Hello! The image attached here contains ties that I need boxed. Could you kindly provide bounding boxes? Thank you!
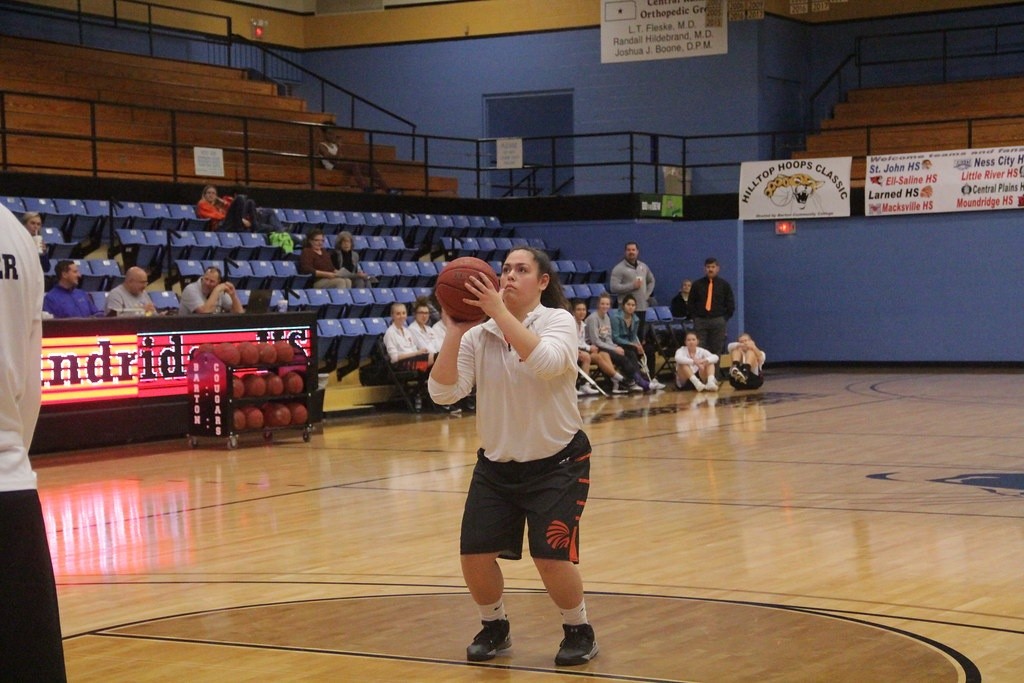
[706,278,713,311]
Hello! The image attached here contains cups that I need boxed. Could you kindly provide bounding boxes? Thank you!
[278,300,288,313]
[33,235,43,255]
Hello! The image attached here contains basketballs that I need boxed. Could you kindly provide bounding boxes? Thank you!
[435,256,499,323]
[199,341,294,366]
[233,400,308,430]
[232,372,304,397]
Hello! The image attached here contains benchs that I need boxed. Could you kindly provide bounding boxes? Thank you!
[790,74,1024,189]
[0,36,461,196]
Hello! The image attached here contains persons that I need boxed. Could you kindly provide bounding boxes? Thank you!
[196,184,284,233]
[318,121,404,195]
[727,333,766,390]
[675,331,719,392]
[20,211,51,273]
[104,267,158,317]
[298,229,370,289]
[178,267,244,315]
[383,299,475,414]
[428,244,600,666]
[610,243,655,345]
[42,260,104,318]
[572,292,666,396]
[670,258,735,387]
[0,201,69,683]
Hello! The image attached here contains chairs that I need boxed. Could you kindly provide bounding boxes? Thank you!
[0,195,694,413]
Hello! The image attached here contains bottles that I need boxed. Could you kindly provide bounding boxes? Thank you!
[415,394,422,413]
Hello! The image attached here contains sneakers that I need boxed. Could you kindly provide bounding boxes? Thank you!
[467,614,511,662]
[554,623,599,666]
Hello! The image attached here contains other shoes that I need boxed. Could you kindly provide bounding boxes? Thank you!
[735,371,746,386]
[612,385,628,393]
[464,399,475,409]
[629,383,643,391]
[620,377,635,387]
[705,381,718,392]
[651,378,665,389]
[579,382,599,395]
[696,381,705,392]
[648,381,658,390]
[444,405,462,413]
[575,391,585,396]
[730,366,739,378]
